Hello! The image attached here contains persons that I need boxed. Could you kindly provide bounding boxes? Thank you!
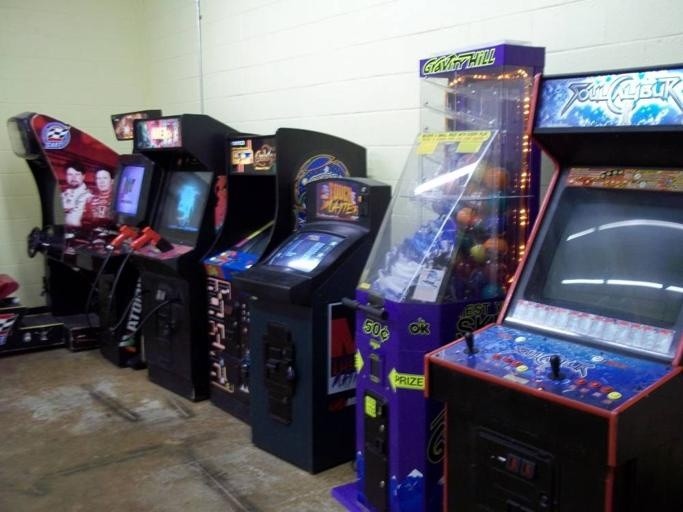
[61,159,93,227]
[81,167,114,226]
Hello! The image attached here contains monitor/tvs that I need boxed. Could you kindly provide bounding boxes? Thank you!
[260,224,364,281]
[51,179,65,225]
[109,163,149,228]
[519,183,683,331]
[152,168,214,246]
